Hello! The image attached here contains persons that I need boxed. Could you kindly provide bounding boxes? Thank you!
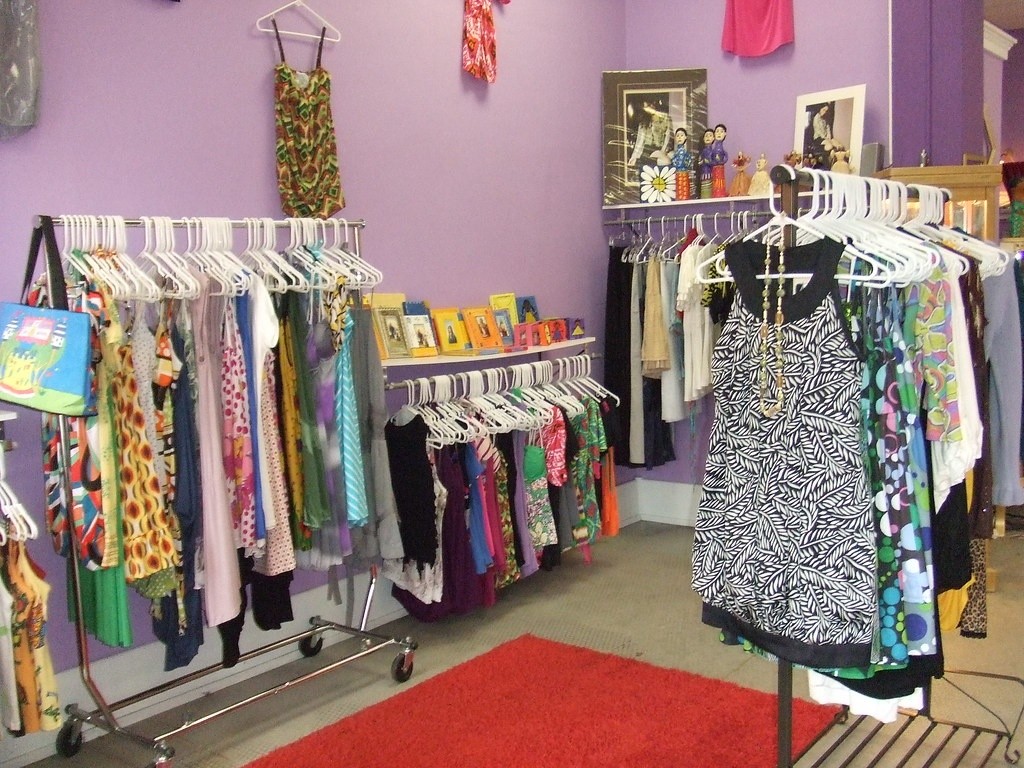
[748,152,774,194]
[627,93,674,168]
[448,325,457,343]
[730,150,753,196]
[674,128,691,201]
[811,102,844,170]
[697,124,728,199]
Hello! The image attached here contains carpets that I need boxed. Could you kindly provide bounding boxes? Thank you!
[243,629,853,768]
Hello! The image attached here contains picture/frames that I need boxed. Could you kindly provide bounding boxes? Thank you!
[599,67,710,201]
[792,85,868,186]
[365,291,586,359]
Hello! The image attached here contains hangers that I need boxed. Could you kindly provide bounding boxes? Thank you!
[46,215,383,304]
[0,443,40,548]
[257,2,343,43]
[607,156,1010,288]
[386,346,624,448]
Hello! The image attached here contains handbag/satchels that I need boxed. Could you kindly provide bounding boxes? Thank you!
[0,216,98,417]
